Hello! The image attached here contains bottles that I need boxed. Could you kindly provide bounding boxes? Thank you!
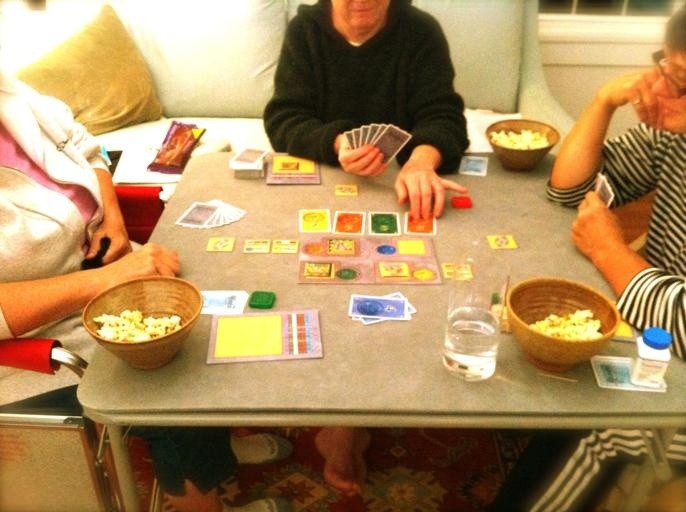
[629,328,672,387]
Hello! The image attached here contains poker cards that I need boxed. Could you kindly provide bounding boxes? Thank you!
[230,146,269,170]
[300,209,437,237]
[199,291,250,315]
[346,122,414,165]
[590,355,668,395]
[460,156,489,176]
[596,172,615,208]
[348,293,417,326]
[175,199,247,229]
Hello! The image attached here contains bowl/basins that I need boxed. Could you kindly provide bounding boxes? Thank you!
[486,118,560,172]
[81,275,204,369]
[505,275,620,373]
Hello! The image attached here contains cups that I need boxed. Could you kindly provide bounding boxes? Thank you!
[443,289,499,381]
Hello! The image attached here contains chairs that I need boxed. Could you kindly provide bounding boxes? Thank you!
[594,459,686,512]
[0,188,166,512]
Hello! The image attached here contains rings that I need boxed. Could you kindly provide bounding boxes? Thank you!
[633,99,644,106]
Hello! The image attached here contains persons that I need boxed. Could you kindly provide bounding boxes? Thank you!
[0,66,297,511]
[262,1,472,495]
[478,3,686,510]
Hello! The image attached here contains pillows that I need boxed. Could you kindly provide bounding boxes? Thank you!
[112,0,288,117]
[289,0,527,112]
[14,0,163,137]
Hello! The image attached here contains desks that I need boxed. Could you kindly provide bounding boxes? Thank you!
[74,151,686,512]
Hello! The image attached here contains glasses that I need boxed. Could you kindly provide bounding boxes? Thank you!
[652,48,685,100]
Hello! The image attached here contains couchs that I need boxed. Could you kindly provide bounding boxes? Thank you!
[0,0,578,203]
